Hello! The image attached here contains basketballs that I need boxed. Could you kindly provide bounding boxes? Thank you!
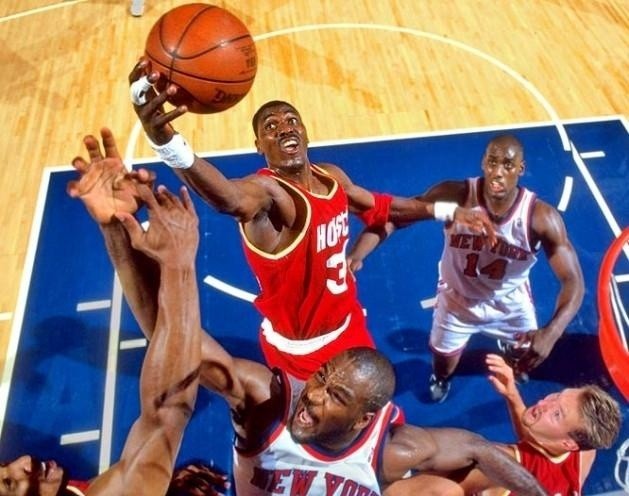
[145,4,257,114]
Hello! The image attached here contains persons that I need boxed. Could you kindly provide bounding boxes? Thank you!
[126,55,500,387]
[68,129,550,496]
[457,352,623,496]
[76,179,206,496]
[1,455,232,496]
[346,133,589,405]
[129,0,146,16]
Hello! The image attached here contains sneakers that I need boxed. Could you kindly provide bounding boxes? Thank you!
[496,339,529,384]
[428,371,451,403]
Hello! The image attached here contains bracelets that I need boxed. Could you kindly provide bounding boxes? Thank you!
[145,129,194,170]
[432,200,459,224]
